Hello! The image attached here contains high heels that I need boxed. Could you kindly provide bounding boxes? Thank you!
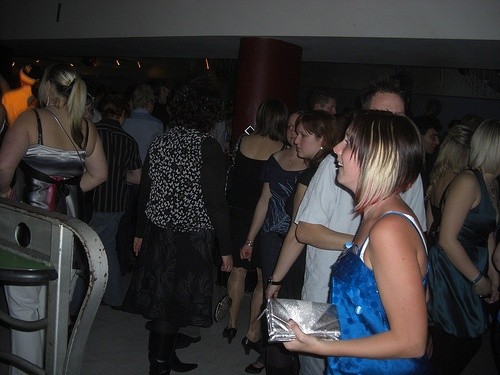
[241,337,262,355]
[223,326,237,344]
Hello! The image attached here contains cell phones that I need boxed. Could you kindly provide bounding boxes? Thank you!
[245,126,255,135]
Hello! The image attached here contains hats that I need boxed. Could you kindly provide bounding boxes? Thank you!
[20,64,42,85]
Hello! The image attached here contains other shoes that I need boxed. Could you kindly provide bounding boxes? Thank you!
[175,333,202,349]
[170,353,198,372]
[245,362,264,373]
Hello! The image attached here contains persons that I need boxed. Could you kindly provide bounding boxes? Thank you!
[294,78,427,374]
[239,107,310,375]
[305,87,338,115]
[284,110,436,374]
[132,75,233,374]
[425,116,500,375]
[0,46,233,284]
[0,60,108,375]
[411,114,440,154]
[414,99,444,124]
[420,116,485,240]
[67,89,140,348]
[224,95,293,355]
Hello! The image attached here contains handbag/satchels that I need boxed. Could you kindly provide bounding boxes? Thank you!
[215,270,232,322]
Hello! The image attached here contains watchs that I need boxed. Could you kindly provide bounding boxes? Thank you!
[267,276,282,285]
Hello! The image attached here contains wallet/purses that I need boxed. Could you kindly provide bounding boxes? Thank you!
[257,299,341,342]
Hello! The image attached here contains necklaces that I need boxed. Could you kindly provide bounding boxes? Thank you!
[489,190,497,198]
[344,193,398,249]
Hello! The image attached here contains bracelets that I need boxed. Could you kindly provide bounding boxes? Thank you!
[470,272,483,285]
